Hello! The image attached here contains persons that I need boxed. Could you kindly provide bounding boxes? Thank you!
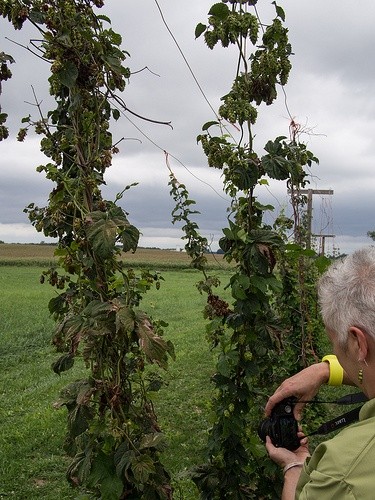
[263,243,375,500]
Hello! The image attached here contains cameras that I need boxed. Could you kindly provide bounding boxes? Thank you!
[257,395,301,452]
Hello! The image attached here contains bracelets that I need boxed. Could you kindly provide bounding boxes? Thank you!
[282,461,305,476]
[320,354,344,386]
